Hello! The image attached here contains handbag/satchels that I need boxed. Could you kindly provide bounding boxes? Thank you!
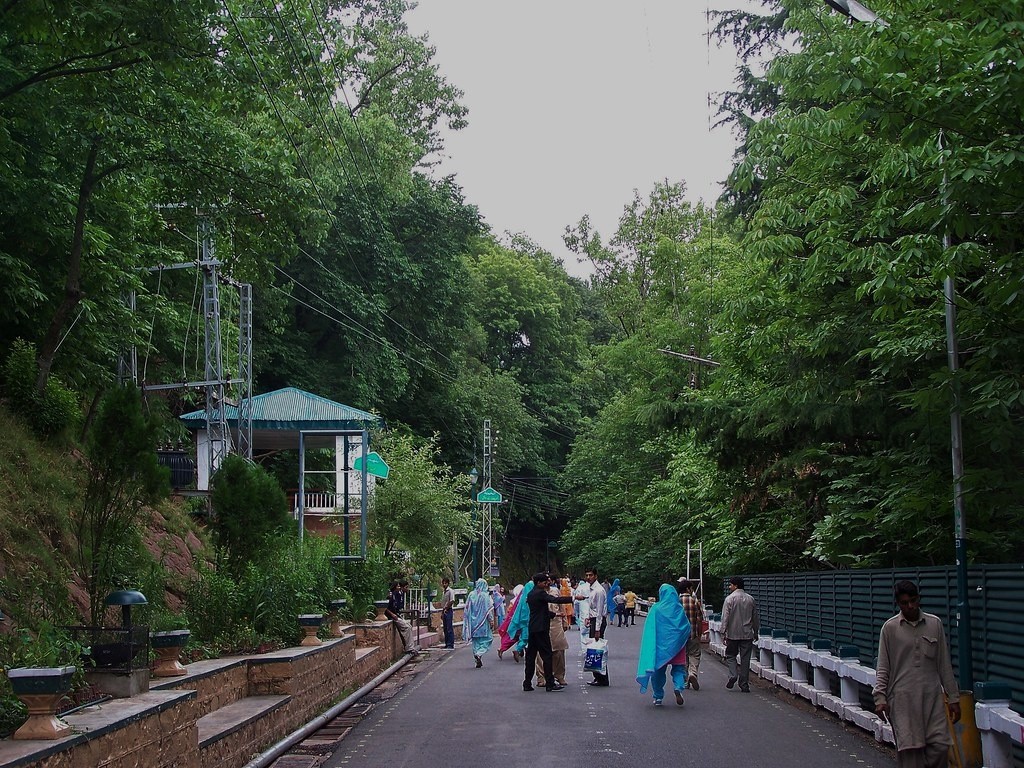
[582,637,608,675]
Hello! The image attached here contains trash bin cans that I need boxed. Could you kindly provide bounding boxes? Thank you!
[700,621,709,644]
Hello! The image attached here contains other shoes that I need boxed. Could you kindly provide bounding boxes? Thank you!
[523,687,534,691]
[689,674,699,691]
[610,621,636,627]
[653,699,662,705]
[440,647,454,650]
[552,684,565,691]
[726,679,735,689]
[537,681,546,687]
[512,650,519,663]
[520,649,524,657]
[554,673,567,685]
[742,687,750,693]
[685,683,690,689]
[474,654,483,669]
[587,681,599,686]
[674,689,684,705]
[497,650,503,660]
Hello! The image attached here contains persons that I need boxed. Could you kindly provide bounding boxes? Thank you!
[674,580,704,692]
[873,582,962,768]
[440,578,456,650]
[721,577,759,692]
[465,567,642,693]
[385,581,420,655]
[643,583,690,707]
